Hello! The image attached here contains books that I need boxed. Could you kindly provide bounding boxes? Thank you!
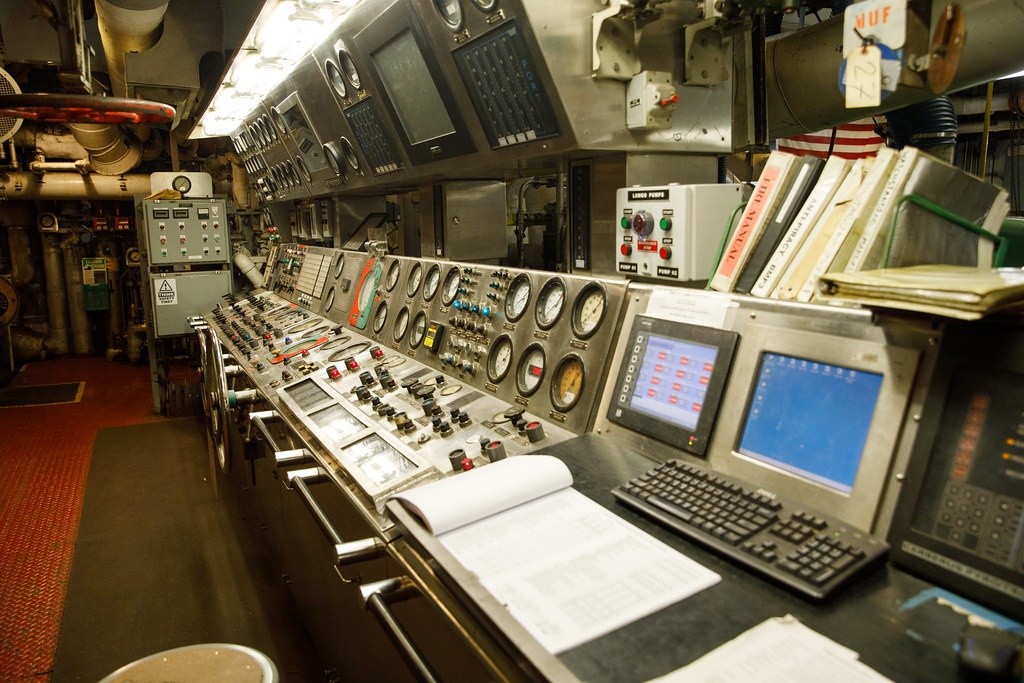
[711,149,1024,321]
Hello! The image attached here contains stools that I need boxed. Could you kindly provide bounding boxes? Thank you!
[97,643,279,683]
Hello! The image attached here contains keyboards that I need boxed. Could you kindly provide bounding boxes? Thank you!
[610,458,891,600]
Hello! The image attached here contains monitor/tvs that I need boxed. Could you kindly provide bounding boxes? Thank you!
[606,314,739,455]
[708,323,921,534]
